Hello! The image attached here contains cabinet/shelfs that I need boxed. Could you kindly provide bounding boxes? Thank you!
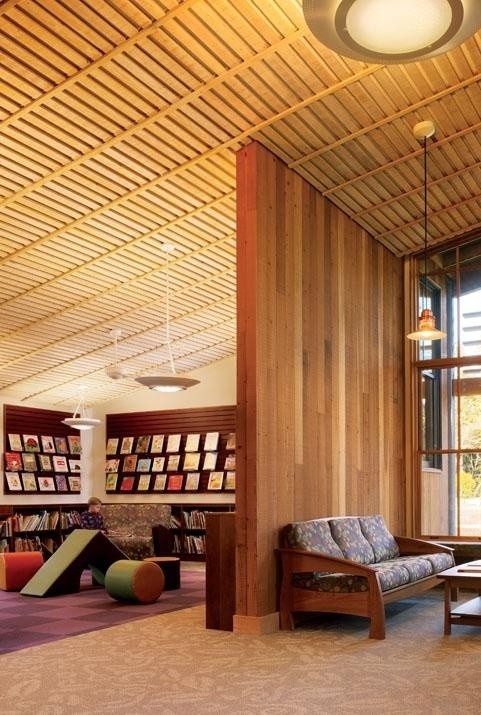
[170,503,236,561]
[0,503,87,565]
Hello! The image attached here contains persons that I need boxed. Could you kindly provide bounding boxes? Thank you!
[81,496,109,534]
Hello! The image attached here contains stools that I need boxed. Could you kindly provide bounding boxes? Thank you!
[144,557,182,589]
[105,559,166,606]
[1,551,45,591]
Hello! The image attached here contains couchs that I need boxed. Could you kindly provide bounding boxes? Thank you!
[97,504,171,560]
[273,517,459,641]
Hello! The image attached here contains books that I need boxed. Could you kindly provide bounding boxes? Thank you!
[6,432,236,492]
[169,504,211,555]
[0,505,80,552]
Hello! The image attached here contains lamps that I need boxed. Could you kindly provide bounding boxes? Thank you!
[405,121,449,340]
[302,1,480,67]
[134,243,201,394]
[106,329,128,381]
[61,383,100,430]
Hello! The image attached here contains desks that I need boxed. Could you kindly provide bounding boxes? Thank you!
[436,557,481,639]
[206,510,237,632]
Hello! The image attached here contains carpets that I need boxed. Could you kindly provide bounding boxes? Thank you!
[0,566,206,654]
